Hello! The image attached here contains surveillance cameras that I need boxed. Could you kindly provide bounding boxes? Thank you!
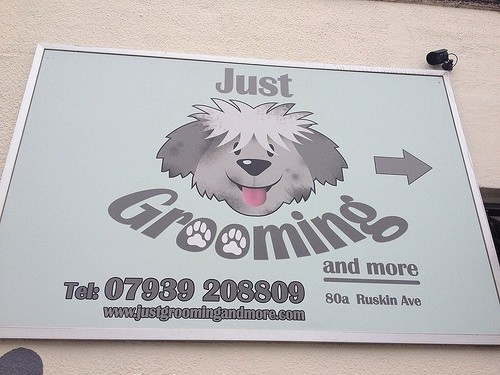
[426,48,454,71]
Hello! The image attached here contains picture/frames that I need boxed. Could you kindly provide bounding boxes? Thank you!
[0,43,500,347]
